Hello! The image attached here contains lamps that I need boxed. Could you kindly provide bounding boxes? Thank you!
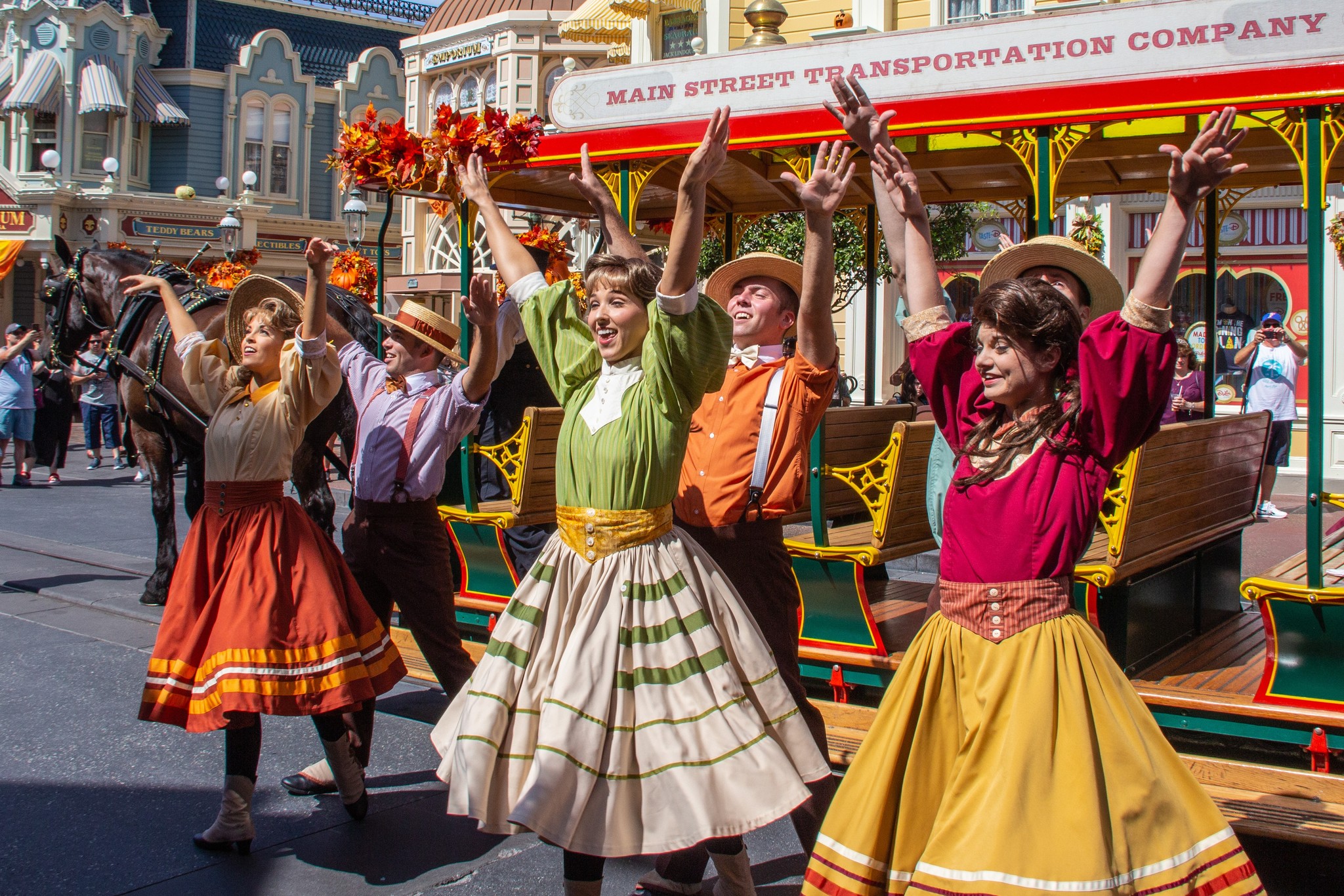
[41,149,61,174]
[102,157,119,178]
[215,176,230,196]
[242,170,257,190]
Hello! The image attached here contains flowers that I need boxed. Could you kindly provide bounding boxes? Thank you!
[328,247,377,304]
[321,99,587,315]
[106,240,263,287]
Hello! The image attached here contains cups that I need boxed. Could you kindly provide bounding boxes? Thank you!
[1170,393,1182,412]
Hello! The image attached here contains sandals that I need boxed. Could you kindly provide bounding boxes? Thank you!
[20,471,32,481]
[49,472,62,484]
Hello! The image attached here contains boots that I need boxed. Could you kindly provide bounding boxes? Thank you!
[193,773,259,855]
[704,833,756,896]
[318,726,371,822]
[563,876,604,896]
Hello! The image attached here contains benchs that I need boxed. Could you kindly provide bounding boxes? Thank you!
[436,407,565,603]
[1069,409,1273,677]
[1240,490,1344,712]
[782,401,917,525]
[783,421,936,656]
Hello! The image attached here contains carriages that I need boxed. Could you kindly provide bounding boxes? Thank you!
[37,0,1344,850]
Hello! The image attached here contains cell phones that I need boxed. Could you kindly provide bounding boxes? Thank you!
[32,323,40,333]
[1261,331,1278,339]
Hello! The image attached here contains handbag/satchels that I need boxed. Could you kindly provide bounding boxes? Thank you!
[33,386,44,409]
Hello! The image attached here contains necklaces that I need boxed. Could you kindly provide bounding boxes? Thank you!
[1174,379,1181,386]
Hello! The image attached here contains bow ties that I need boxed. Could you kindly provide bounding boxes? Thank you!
[728,345,761,370]
[386,374,408,394]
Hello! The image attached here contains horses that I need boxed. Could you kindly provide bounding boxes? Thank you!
[39,233,390,604]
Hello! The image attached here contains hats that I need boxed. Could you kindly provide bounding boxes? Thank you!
[6,323,27,334]
[976,233,1126,336]
[1262,312,1282,323]
[224,273,305,367]
[371,299,469,366]
[489,243,550,278]
[704,250,807,339]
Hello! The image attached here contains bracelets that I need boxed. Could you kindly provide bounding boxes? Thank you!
[1189,402,1194,416]
[1285,339,1292,343]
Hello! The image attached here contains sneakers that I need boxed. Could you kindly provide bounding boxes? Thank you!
[12,475,32,488]
[1256,501,1288,519]
[87,458,102,470]
[133,469,149,483]
[112,458,124,469]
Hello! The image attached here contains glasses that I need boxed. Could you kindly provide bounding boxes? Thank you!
[10,330,25,336]
[89,339,102,344]
[1263,322,1279,329]
[782,352,795,358]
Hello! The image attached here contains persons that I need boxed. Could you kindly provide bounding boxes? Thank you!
[122,238,367,846]
[431,105,830,896]
[569,139,855,896]
[21,327,74,484]
[281,275,501,797]
[1234,312,1307,519]
[71,330,150,483]
[0,323,44,487]
[794,127,1274,896]
[821,73,1249,628]
[1160,335,1218,425]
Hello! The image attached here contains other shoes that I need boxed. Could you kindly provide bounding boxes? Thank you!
[119,448,127,456]
[281,772,368,795]
[87,451,102,460]
[633,882,703,896]
